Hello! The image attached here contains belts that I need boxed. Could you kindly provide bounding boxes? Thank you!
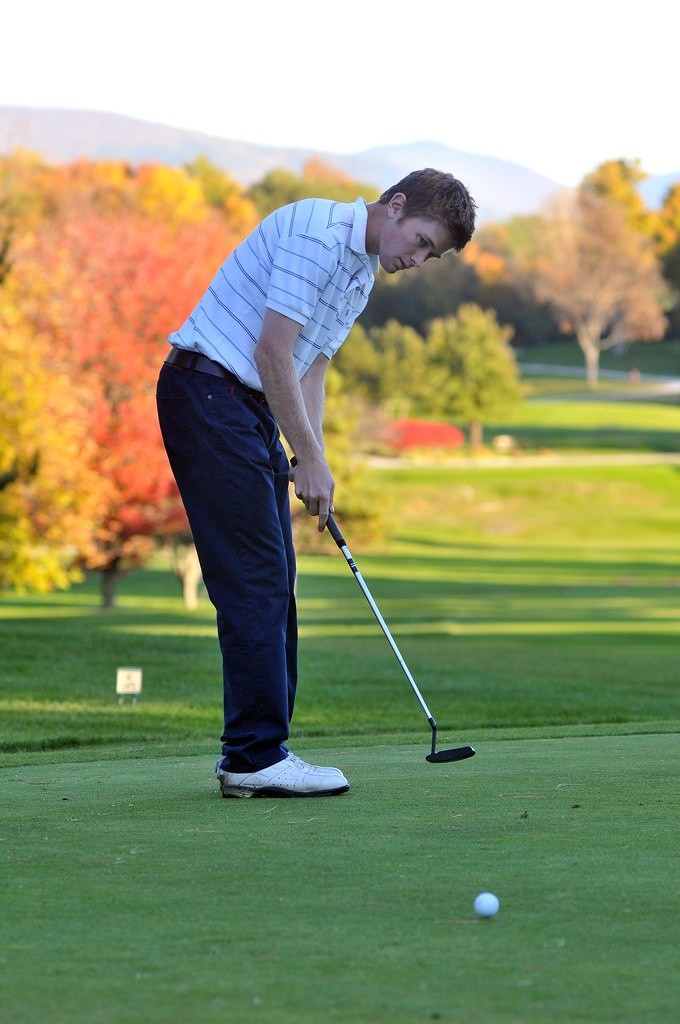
[163,348,235,380]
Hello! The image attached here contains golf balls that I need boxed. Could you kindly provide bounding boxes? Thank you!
[474,893,499,919]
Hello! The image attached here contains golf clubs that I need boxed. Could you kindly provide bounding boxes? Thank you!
[286,450,479,763]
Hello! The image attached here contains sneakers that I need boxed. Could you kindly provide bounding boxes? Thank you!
[215,750,349,798]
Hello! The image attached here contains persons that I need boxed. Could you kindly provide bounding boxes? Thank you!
[153,167,480,797]
